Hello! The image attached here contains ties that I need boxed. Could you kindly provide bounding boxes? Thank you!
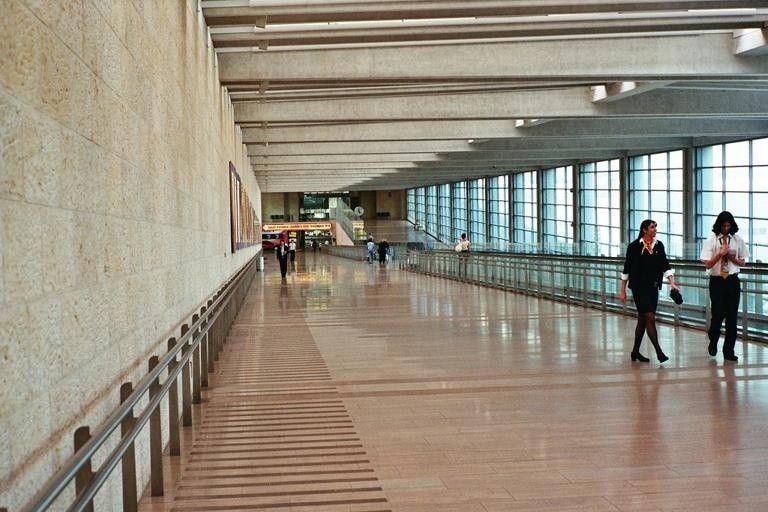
[719,236,730,279]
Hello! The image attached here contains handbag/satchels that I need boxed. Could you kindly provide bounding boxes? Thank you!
[455,241,463,253]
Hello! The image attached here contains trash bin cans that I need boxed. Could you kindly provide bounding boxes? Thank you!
[256,257,264,271]
[407,242,425,268]
[413,224,420,231]
[387,247,395,260]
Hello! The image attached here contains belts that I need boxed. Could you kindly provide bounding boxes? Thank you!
[709,272,739,279]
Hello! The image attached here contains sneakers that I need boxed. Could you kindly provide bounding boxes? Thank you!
[631,352,651,362]
[723,350,739,361]
[708,340,718,356]
[657,354,670,363]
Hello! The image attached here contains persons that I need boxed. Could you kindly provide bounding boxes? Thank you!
[619,219,680,363]
[277,240,290,278]
[407,246,420,270]
[700,211,751,361]
[310,239,317,253]
[458,233,471,277]
[324,239,330,246]
[367,238,376,264]
[378,239,387,265]
[288,240,297,261]
